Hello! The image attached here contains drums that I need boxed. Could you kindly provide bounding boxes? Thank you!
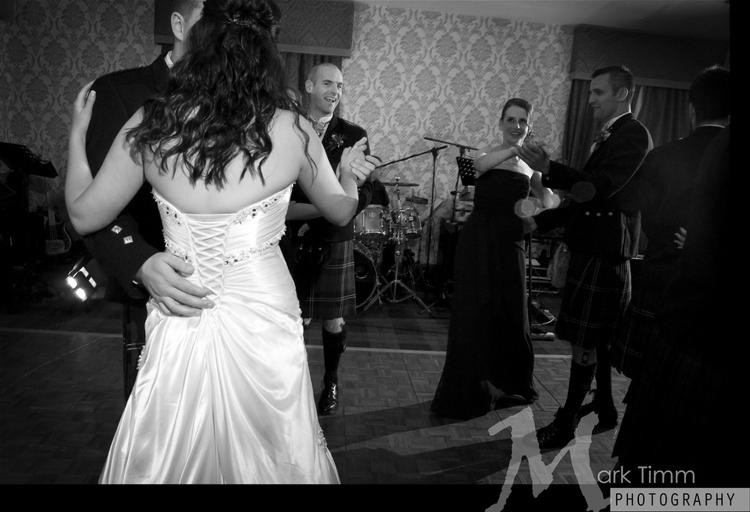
[353,238,377,308]
[352,204,390,240]
[391,207,422,240]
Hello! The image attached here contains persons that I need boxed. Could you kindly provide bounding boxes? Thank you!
[599,67,731,496]
[355,156,390,206]
[78,0,207,400]
[430,98,556,421]
[65,0,366,486]
[513,63,654,450]
[671,125,731,300]
[279,62,369,416]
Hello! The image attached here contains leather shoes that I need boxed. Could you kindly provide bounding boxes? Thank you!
[522,406,577,454]
[575,398,618,427]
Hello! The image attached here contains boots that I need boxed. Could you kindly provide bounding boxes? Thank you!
[318,327,347,415]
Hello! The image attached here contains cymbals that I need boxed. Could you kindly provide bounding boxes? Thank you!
[382,183,420,186]
